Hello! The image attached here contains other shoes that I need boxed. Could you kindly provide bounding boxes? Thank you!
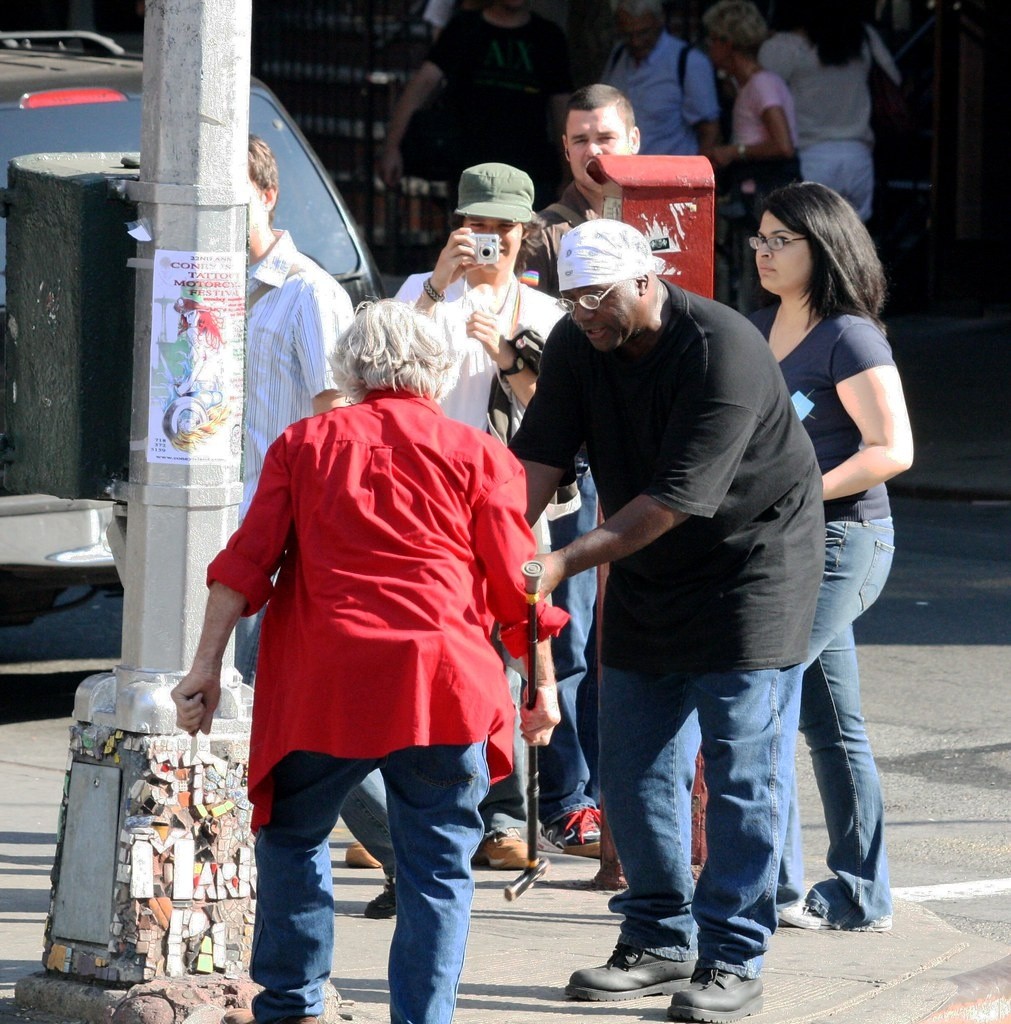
[778,903,893,933]
[220,1009,320,1024]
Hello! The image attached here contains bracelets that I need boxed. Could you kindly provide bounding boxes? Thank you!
[423,277,445,302]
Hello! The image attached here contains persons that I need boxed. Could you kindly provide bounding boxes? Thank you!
[171,300,568,1024]
[508,217,825,1024]
[736,182,914,935]
[595,0,899,315]
[342,163,582,870]
[235,133,404,920]
[511,78,644,858]
[375,0,582,212]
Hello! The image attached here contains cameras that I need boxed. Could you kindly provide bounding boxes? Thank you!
[461,234,500,264]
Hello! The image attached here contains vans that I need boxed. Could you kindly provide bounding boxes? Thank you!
[0,29,391,687]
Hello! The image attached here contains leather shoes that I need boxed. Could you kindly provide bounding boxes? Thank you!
[666,968,763,1023]
[564,945,697,1001]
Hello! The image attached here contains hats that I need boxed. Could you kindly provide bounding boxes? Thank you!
[455,163,534,223]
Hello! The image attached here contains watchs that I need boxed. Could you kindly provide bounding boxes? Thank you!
[737,144,745,160]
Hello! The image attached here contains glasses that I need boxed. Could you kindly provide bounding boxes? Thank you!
[749,236,809,250]
[556,282,618,314]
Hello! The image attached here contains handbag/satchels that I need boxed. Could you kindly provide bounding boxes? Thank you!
[867,39,921,152]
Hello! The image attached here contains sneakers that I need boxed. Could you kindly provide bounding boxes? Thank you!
[537,806,601,858]
[345,841,382,868]
[473,828,528,870]
[364,878,396,918]
